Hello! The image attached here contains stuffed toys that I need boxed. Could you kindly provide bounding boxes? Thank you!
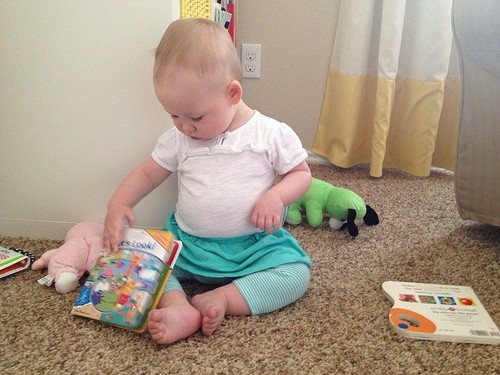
[31,220,105,293]
[285,177,380,238]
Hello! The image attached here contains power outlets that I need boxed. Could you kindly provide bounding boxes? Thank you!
[241,43,262,80]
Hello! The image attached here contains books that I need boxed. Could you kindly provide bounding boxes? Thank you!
[0,246,28,279]
[382,281,500,345]
[70,228,182,333]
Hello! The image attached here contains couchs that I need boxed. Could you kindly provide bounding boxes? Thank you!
[453,23,500,228]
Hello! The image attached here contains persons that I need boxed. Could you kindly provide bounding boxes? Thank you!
[103,18,312,344]
